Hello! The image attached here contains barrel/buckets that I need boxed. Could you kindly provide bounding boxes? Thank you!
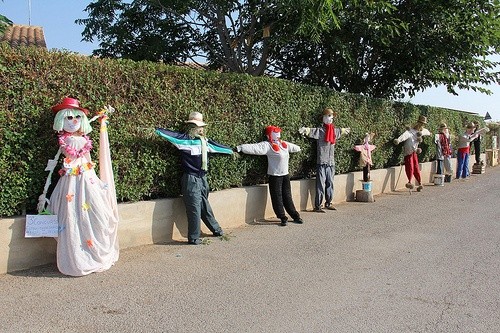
[362,182,372,191]
[433,174,445,187]
